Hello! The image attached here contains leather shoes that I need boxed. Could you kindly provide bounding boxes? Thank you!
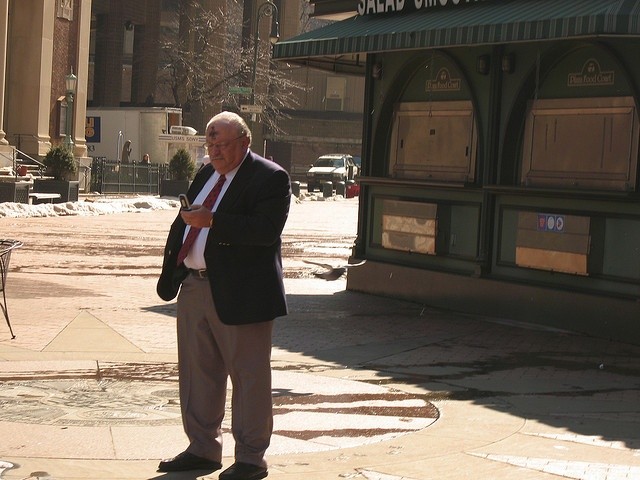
[219,462,268,480]
[158,449,222,471]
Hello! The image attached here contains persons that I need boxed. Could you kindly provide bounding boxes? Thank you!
[122,140,132,163]
[156,111,291,480]
[141,153,150,164]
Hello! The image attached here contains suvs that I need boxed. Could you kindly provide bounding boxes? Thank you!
[307,153,360,192]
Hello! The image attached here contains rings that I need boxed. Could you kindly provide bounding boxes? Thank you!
[186,219,193,226]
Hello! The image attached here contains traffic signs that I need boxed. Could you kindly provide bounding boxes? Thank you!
[228,86,252,94]
[240,105,262,114]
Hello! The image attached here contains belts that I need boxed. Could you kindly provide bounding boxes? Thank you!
[182,266,208,279]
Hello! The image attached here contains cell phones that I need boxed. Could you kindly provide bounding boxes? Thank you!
[179,194,192,210]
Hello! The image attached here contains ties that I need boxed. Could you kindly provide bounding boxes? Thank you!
[176,174,226,267]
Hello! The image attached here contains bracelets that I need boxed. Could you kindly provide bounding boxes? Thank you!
[209,216,214,230]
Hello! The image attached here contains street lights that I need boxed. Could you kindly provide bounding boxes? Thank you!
[249,2,278,132]
[65,65,77,154]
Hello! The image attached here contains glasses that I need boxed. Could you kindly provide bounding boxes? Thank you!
[200,133,246,151]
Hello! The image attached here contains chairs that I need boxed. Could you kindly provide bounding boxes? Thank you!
[1,239,24,339]
